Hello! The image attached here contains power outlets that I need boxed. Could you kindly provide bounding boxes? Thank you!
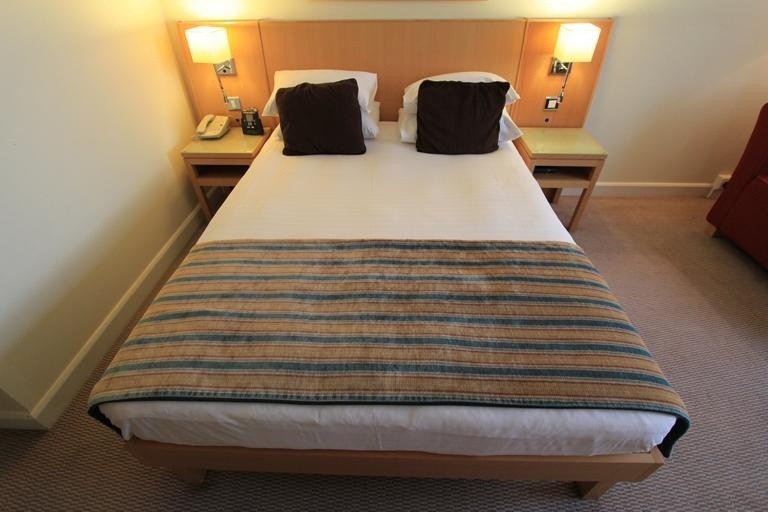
[714,173,735,192]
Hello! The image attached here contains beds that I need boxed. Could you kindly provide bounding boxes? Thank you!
[85,120,691,500]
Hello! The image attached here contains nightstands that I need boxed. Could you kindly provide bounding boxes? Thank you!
[515,127,608,232]
[179,126,271,222]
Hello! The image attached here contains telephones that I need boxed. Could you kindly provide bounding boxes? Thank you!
[191,114,229,141]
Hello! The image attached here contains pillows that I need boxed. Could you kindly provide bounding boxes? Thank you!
[275,78,366,155]
[403,71,520,113]
[398,108,524,143]
[415,79,510,154]
[261,69,378,116]
[274,101,380,139]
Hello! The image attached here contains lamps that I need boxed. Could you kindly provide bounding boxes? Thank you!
[185,25,236,104]
[547,22,601,102]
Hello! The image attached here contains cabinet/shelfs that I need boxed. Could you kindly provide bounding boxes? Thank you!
[706,105,768,272]
